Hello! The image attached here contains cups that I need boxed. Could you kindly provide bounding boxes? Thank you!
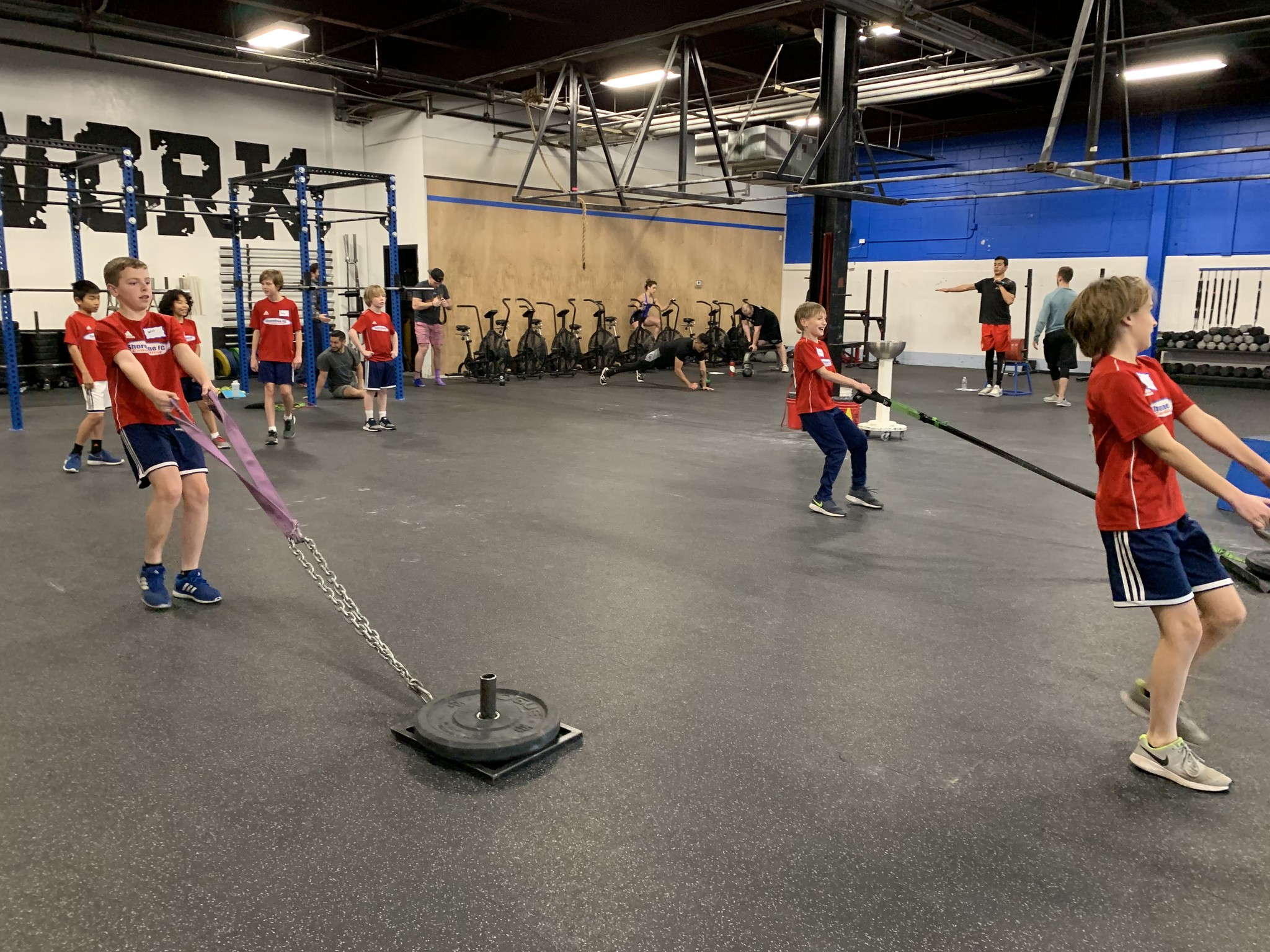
[231,380,240,400]
[499,368,505,386]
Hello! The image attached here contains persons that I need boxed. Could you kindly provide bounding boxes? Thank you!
[94,256,224,609]
[302,330,379,398]
[294,262,331,388]
[792,301,884,518]
[348,284,399,432]
[629,279,675,344]
[740,302,789,373]
[600,333,715,390]
[249,270,302,446]
[1032,267,1079,407]
[158,289,232,450]
[413,267,452,387]
[61,280,125,473]
[1065,274,1270,793]
[935,255,1016,397]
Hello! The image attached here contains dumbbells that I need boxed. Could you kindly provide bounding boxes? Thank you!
[741,361,754,377]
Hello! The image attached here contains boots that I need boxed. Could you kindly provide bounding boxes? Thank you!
[435,369,446,386]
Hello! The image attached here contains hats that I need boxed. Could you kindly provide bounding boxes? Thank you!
[426,268,444,283]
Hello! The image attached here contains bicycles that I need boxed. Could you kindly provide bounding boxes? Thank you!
[450,298,797,382]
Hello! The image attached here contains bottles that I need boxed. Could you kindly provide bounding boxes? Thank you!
[962,377,967,391]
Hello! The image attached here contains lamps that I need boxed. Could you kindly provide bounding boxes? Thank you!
[600,64,682,88]
[245,21,311,49]
[814,28,823,44]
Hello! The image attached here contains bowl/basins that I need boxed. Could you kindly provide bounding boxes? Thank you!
[866,340,906,359]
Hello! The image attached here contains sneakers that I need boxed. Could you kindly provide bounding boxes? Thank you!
[86,449,125,465]
[172,568,220,603]
[781,365,789,373]
[846,485,882,510]
[62,452,81,472]
[377,416,396,430]
[1043,394,1060,403]
[808,493,848,518]
[635,370,645,382]
[1128,730,1234,794]
[600,367,611,385]
[134,566,171,609]
[988,384,1003,397]
[978,384,991,396]
[414,378,426,387]
[283,414,296,439]
[265,430,278,444]
[363,417,382,431]
[1056,398,1072,407]
[210,436,232,449]
[1118,677,1211,746]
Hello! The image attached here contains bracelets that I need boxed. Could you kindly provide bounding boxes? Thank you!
[431,302,434,306]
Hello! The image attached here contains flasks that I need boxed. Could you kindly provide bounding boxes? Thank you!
[729,358,735,377]
